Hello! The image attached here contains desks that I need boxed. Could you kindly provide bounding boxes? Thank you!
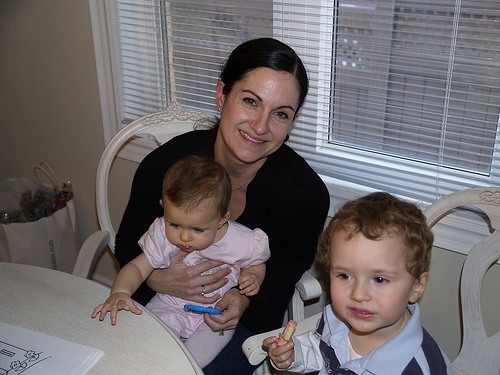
[0,262,205,374]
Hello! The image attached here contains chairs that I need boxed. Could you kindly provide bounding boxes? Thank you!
[240,187,500,374]
[71,106,219,278]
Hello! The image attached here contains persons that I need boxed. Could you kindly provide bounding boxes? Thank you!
[114,38,331,375]
[91,157,270,370]
[263,192,452,375]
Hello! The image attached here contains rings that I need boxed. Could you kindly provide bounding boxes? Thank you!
[202,285,206,296]
[219,329,224,336]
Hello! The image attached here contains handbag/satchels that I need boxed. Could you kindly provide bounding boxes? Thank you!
[3,161,81,274]
